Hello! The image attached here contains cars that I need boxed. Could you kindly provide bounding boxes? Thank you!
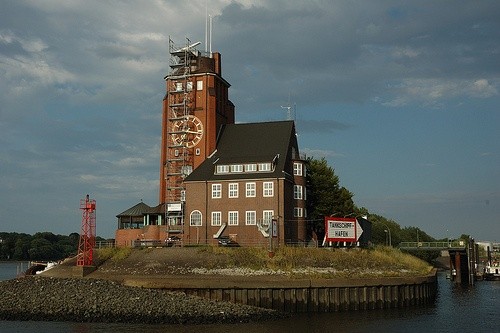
[165,236,182,243]
[218,235,233,247]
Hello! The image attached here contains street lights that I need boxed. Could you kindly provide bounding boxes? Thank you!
[416,226,419,247]
[383,230,389,245]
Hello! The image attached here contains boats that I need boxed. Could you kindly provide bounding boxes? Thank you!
[484,262,500,281]
[446,274,451,279]
